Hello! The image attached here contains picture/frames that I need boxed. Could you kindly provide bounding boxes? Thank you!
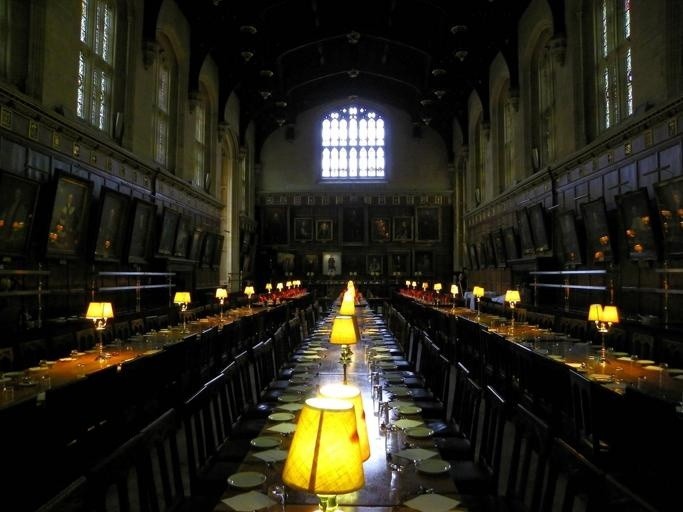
[0,167,215,269]
[468,175,682,270]
[259,203,443,280]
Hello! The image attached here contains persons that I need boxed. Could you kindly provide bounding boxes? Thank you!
[0,188,117,267]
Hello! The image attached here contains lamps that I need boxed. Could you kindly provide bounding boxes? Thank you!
[215,288,228,319]
[172,291,193,335]
[240,24,467,125]
[277,282,283,294]
[504,290,521,325]
[587,303,619,351]
[244,286,255,309]
[84,301,114,359]
[473,286,485,312]
[285,280,301,291]
[434,283,442,294]
[422,282,428,291]
[405,281,411,288]
[328,281,360,380]
[412,282,417,290]
[265,283,273,295]
[450,285,458,304]
[281,382,373,511]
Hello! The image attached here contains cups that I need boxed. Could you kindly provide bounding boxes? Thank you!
[3,385,14,400]
[38,375,49,389]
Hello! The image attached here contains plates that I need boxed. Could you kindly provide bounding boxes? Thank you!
[249,436,282,448]
[268,412,295,421]
[227,471,266,490]
[278,394,302,402]
[415,290,670,402]
[399,405,422,415]
[0,303,251,386]
[415,458,451,476]
[406,427,434,438]
[282,290,413,395]
